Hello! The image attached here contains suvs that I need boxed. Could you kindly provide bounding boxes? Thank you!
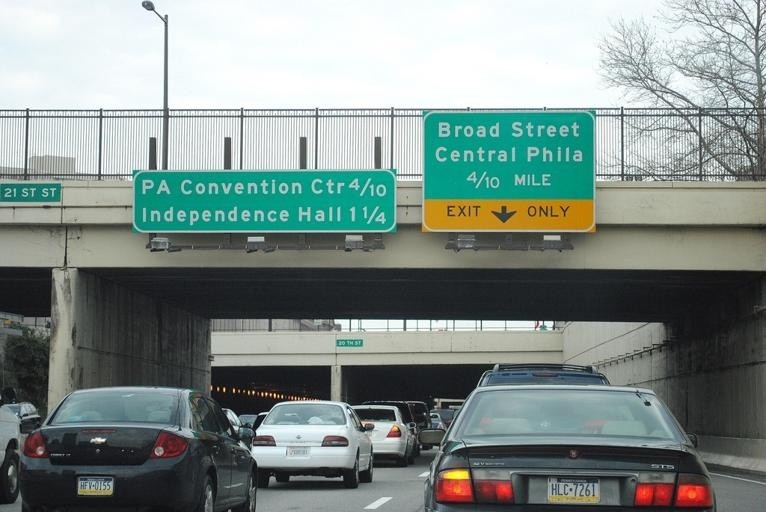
[4,401,41,433]
[0,395,20,503]
[475,363,612,386]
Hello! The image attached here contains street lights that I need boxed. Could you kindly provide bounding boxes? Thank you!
[141,0,169,170]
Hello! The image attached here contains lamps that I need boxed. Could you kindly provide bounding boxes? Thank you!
[150,237,173,252]
[456,233,476,250]
[246,236,266,253]
[345,234,364,252]
[543,234,564,249]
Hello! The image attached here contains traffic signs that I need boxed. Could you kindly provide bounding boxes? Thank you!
[424,110,597,234]
[0,182,61,203]
[131,166,400,237]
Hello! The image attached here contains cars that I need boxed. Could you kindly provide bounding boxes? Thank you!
[416,385,717,511]
[219,408,241,439]
[405,400,434,450]
[433,408,455,430]
[423,412,447,441]
[250,400,374,489]
[350,405,416,467]
[19,388,258,510]
[361,400,420,456]
[254,410,268,434]
[237,414,255,428]
